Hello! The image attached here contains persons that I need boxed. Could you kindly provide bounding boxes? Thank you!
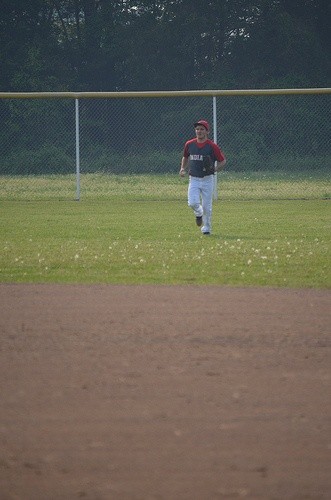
[179,119,226,233]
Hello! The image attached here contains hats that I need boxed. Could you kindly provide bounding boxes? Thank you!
[194,120,210,130]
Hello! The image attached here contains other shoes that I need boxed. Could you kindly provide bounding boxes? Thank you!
[201,224,210,233]
[196,216,203,226]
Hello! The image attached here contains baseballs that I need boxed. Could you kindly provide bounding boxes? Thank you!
[182,171,186,175]
